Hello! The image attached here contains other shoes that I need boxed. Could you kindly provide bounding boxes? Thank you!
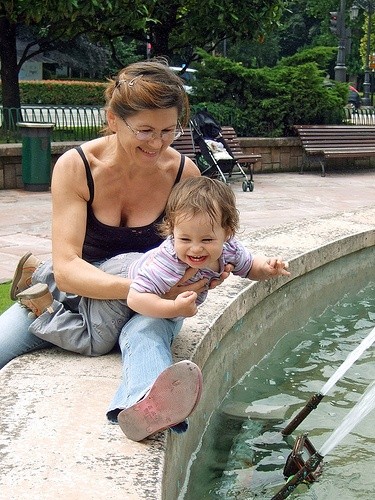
[117,360,203,442]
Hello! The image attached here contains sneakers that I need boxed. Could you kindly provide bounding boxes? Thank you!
[10,250,42,301]
[16,283,54,316]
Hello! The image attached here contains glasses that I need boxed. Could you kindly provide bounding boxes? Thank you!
[121,116,184,142]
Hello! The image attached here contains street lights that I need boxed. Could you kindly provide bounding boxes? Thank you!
[350,0,375,106]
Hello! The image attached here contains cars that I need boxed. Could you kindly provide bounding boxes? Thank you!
[349,86,360,115]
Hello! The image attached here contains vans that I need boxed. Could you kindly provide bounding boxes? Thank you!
[170,67,197,95]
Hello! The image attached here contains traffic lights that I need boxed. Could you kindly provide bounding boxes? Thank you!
[328,7,340,39]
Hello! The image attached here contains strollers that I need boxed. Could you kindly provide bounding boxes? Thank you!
[189,111,254,192]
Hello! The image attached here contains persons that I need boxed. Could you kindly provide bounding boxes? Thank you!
[10,176,292,356]
[1,59,233,442]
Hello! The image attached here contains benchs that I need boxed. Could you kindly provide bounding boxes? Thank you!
[171,126,263,182]
[289,125,375,177]
